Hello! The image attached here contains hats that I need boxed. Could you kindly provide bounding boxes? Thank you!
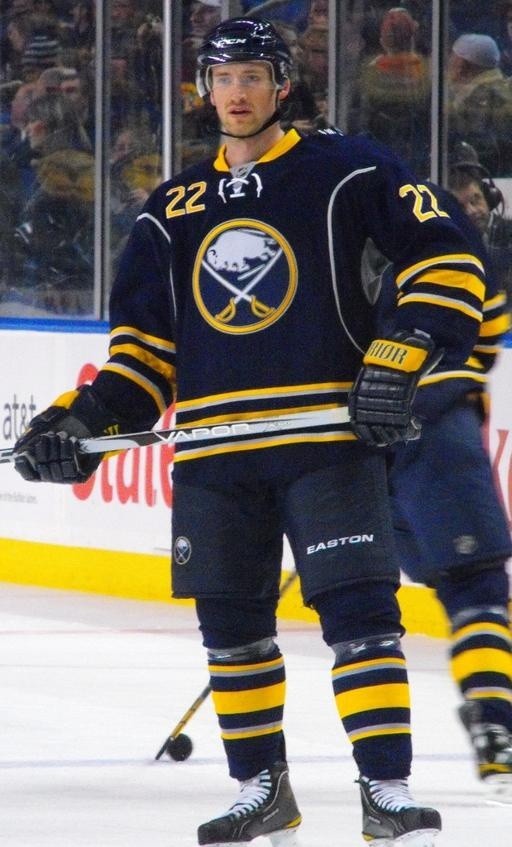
[454,32,501,69]
[381,8,419,44]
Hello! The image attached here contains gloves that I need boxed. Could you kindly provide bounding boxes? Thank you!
[347,328,446,448]
[12,383,129,483]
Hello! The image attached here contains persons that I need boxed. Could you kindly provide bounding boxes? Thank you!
[311,217,511,788]
[2,1,512,335]
[3,15,494,847]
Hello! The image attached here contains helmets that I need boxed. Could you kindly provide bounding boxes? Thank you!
[196,15,294,97]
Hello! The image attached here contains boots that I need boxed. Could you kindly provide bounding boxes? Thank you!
[459,699,510,778]
[358,771,442,840]
[198,759,301,844]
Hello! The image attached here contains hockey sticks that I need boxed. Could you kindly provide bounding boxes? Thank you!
[153,567,299,765]
